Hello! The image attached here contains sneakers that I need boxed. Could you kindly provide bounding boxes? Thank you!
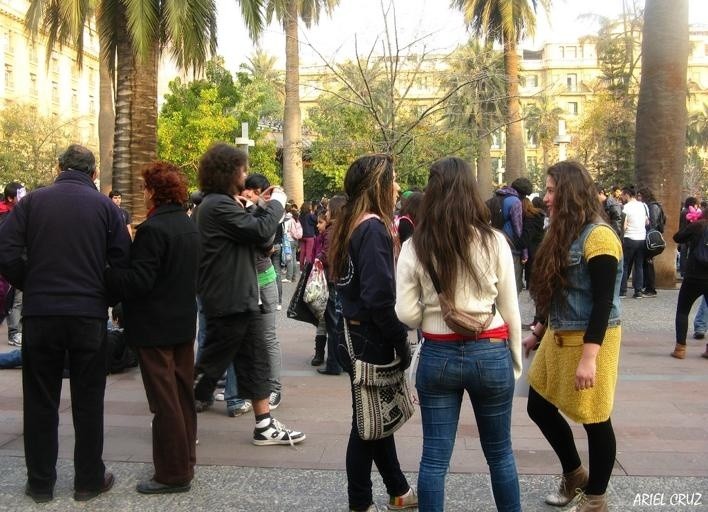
[229,399,253,416]
[252,417,305,446]
[281,278,291,283]
[633,292,642,299]
[388,486,419,509]
[546,466,590,506]
[642,289,657,298]
[215,388,225,400]
[268,392,281,410]
[564,494,609,512]
[671,342,686,359]
[195,397,214,412]
[7,333,22,346]
[619,291,626,298]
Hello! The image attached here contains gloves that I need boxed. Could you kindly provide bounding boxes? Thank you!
[393,333,411,370]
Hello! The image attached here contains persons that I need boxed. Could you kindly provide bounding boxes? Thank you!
[107,190,133,242]
[678,196,699,280]
[299,199,319,273]
[239,173,283,411]
[521,198,548,289]
[480,177,531,297]
[110,163,199,495]
[618,183,650,300]
[593,181,622,238]
[524,160,624,512]
[670,200,708,360]
[0,144,145,504]
[190,144,308,446]
[393,157,523,512]
[320,154,419,512]
[282,204,302,283]
[397,192,424,247]
[635,188,666,299]
[315,195,347,376]
[0,181,25,348]
[316,210,328,235]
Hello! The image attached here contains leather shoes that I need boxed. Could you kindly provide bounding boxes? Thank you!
[74,472,114,502]
[25,479,53,503]
[317,363,341,375]
[136,478,190,494]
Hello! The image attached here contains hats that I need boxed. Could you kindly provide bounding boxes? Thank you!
[58,143,95,173]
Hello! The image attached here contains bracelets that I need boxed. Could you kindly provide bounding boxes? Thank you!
[531,330,542,342]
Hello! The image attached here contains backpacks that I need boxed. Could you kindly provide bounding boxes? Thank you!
[290,217,303,241]
[482,194,516,233]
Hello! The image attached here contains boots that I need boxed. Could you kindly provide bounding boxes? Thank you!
[311,335,327,366]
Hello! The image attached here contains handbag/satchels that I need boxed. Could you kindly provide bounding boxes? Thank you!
[421,255,496,338]
[643,205,666,258]
[287,260,319,327]
[335,293,415,440]
[303,257,330,321]
